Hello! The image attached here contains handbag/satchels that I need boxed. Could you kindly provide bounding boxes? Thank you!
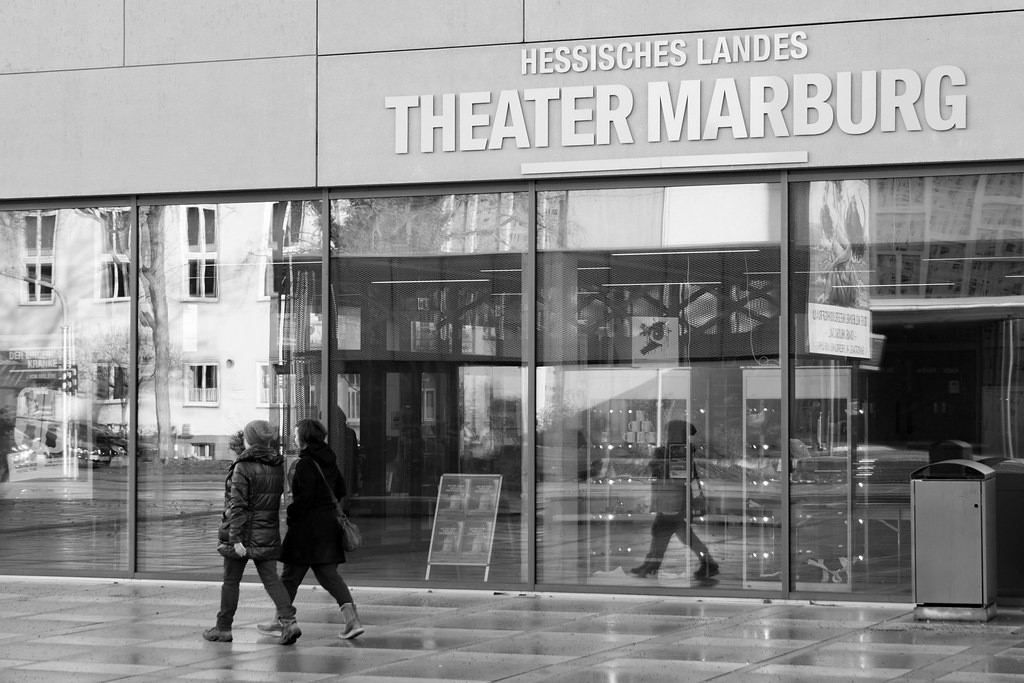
[690,492,710,517]
[336,514,362,552]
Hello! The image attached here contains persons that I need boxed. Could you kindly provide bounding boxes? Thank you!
[257,419,364,640]
[204,420,301,646]
[630,419,720,580]
[0,408,14,482]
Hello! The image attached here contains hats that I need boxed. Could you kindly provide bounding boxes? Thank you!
[666,420,697,443]
[244,420,278,448]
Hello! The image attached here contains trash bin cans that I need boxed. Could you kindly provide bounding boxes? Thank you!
[929,440,972,480]
[909,459,997,622]
[977,456,1024,608]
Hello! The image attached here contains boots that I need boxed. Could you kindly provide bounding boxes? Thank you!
[693,553,719,579]
[256,605,283,636]
[631,565,658,578]
[339,602,364,639]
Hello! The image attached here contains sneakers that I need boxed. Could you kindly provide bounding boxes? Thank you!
[280,619,302,646]
[202,626,232,642]
